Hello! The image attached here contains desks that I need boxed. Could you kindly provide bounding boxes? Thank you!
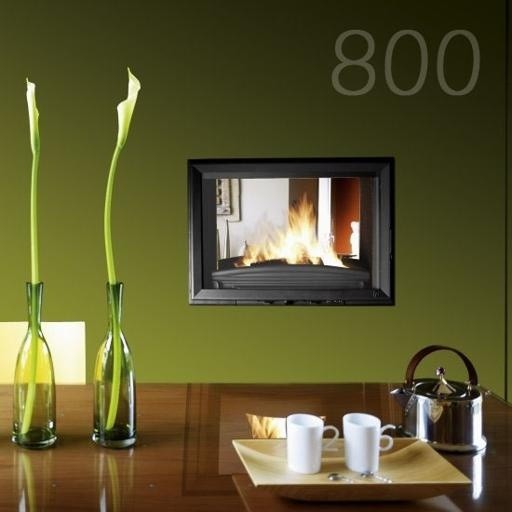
[1,384,511,512]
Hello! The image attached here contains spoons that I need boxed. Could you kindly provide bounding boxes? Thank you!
[361,472,392,483]
[328,473,360,485]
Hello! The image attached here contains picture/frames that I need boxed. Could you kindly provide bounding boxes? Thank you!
[186,158,395,307]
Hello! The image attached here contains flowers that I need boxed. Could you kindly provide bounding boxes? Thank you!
[23,67,142,430]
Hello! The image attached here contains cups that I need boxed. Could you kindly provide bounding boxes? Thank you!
[286,412,340,474]
[342,412,393,473]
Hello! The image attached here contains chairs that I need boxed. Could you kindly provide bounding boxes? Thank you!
[0,321,87,383]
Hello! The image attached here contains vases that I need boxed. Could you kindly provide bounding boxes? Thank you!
[13,282,136,449]
[13,447,134,512]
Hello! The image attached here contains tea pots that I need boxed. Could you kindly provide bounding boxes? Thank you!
[389,344,492,455]
